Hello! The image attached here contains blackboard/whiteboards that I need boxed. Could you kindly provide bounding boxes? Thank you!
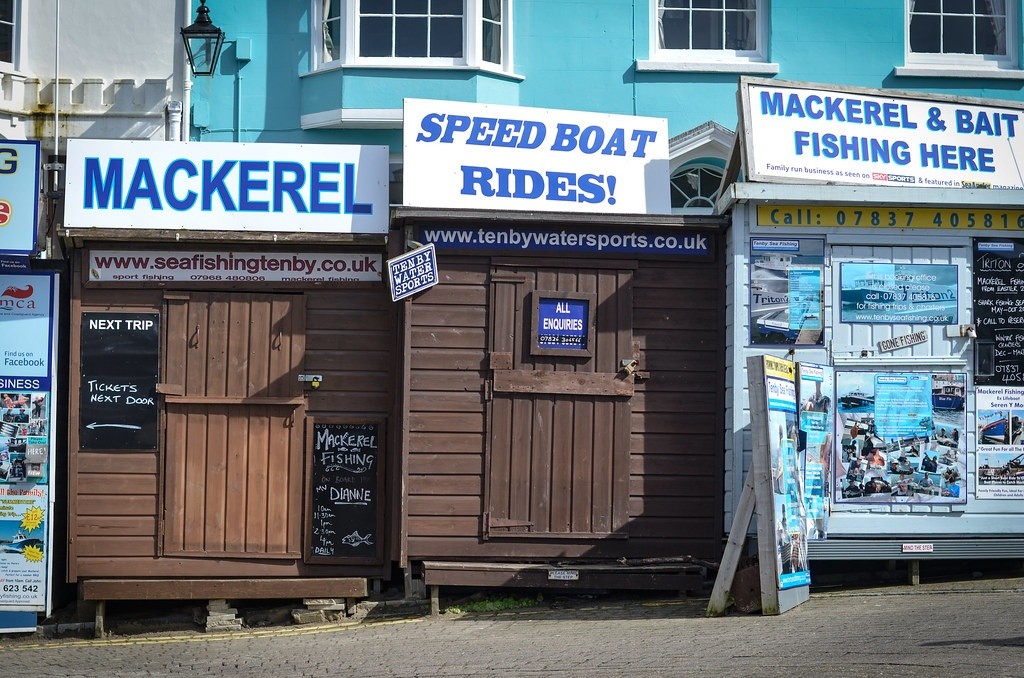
[972,236,1024,386]
[78,312,160,451]
[304,416,386,565]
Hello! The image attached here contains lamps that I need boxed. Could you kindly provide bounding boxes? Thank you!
[180,0,226,79]
